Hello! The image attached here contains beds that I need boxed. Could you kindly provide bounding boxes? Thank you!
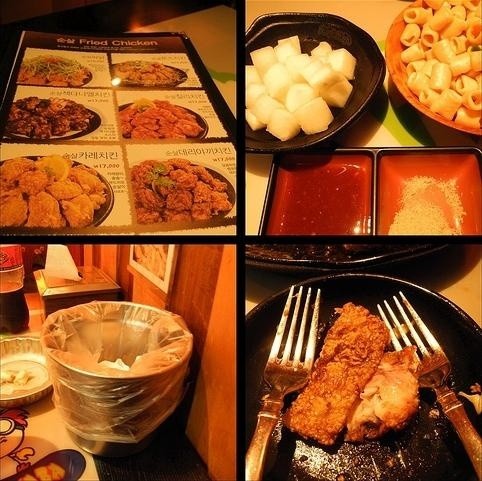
[123,5,237,116]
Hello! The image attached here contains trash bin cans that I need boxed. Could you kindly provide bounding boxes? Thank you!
[39,301,195,458]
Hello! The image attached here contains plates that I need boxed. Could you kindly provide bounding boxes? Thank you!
[3,59,236,227]
[246,272,482,477]
[244,0,482,243]
[1,336,52,409]
[244,241,457,267]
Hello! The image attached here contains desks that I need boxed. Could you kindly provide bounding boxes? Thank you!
[33,265,122,324]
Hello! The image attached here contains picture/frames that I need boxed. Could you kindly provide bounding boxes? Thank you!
[128,244,181,294]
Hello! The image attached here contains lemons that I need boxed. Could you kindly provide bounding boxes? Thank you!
[35,155,70,182]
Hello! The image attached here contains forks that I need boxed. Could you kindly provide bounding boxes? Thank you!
[245,282,325,477]
[377,291,482,477]
